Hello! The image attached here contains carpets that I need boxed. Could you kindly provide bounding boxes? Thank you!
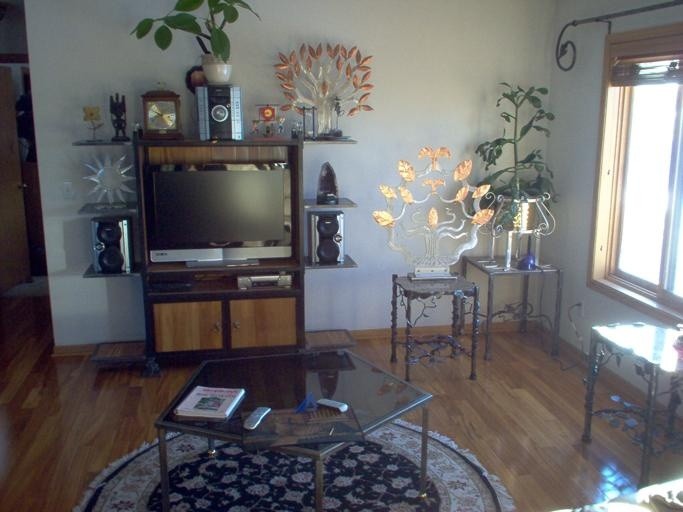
[64,404,519,512]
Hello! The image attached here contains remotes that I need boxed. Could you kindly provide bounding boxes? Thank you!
[243,406,271,430]
[316,398,348,413]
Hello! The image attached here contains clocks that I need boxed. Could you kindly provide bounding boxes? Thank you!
[139,86,185,141]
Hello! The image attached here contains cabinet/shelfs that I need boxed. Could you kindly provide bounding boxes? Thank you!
[85,126,357,371]
[300,131,365,275]
[69,134,143,280]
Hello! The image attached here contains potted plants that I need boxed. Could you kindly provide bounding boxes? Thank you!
[470,79,562,230]
[124,0,264,87]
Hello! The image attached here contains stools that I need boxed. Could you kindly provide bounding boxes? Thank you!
[477,228,556,272]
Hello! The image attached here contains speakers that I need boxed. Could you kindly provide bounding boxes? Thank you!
[91,217,134,274]
[308,211,344,265]
[196,85,243,140]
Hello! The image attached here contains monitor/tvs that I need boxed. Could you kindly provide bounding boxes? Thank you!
[143,163,292,268]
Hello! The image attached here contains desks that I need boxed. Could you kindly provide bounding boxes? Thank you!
[386,272,483,387]
[577,317,682,493]
[463,252,568,366]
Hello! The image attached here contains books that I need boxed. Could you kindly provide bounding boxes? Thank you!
[173,385,245,420]
